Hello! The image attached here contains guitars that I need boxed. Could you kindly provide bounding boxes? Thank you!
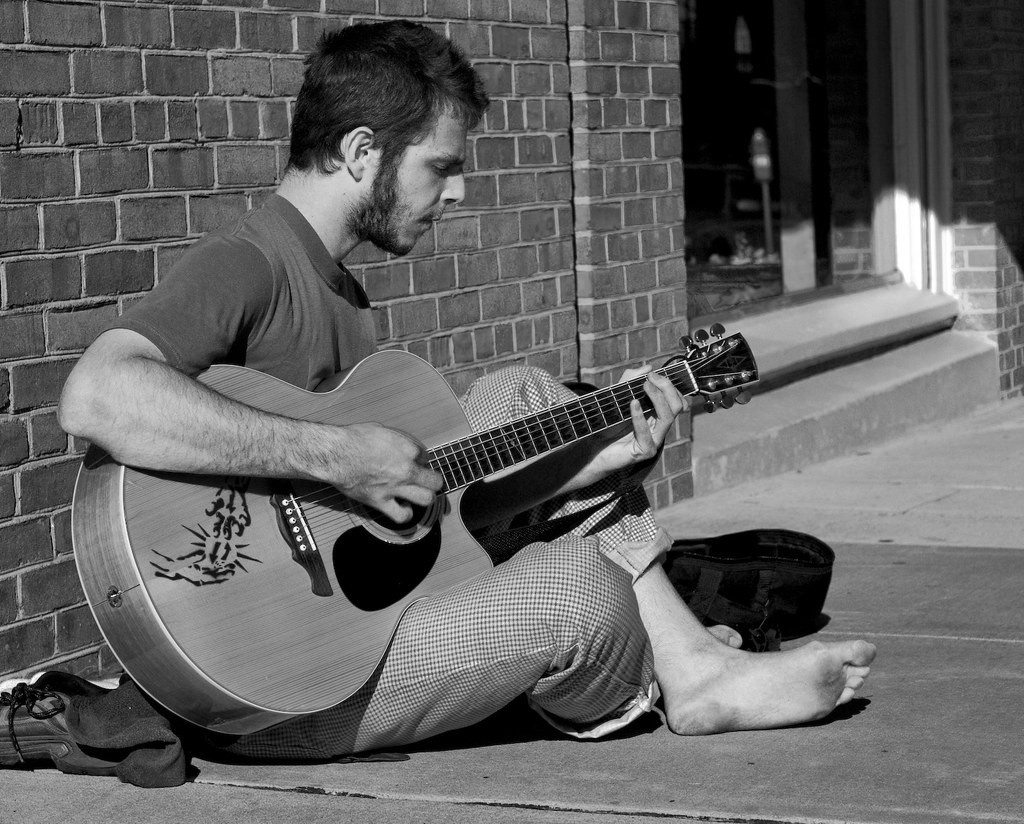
[70,320,764,736]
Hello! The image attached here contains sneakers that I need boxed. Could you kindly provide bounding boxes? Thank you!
[0,682,122,775]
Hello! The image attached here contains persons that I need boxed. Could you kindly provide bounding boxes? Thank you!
[55,19,878,764]
